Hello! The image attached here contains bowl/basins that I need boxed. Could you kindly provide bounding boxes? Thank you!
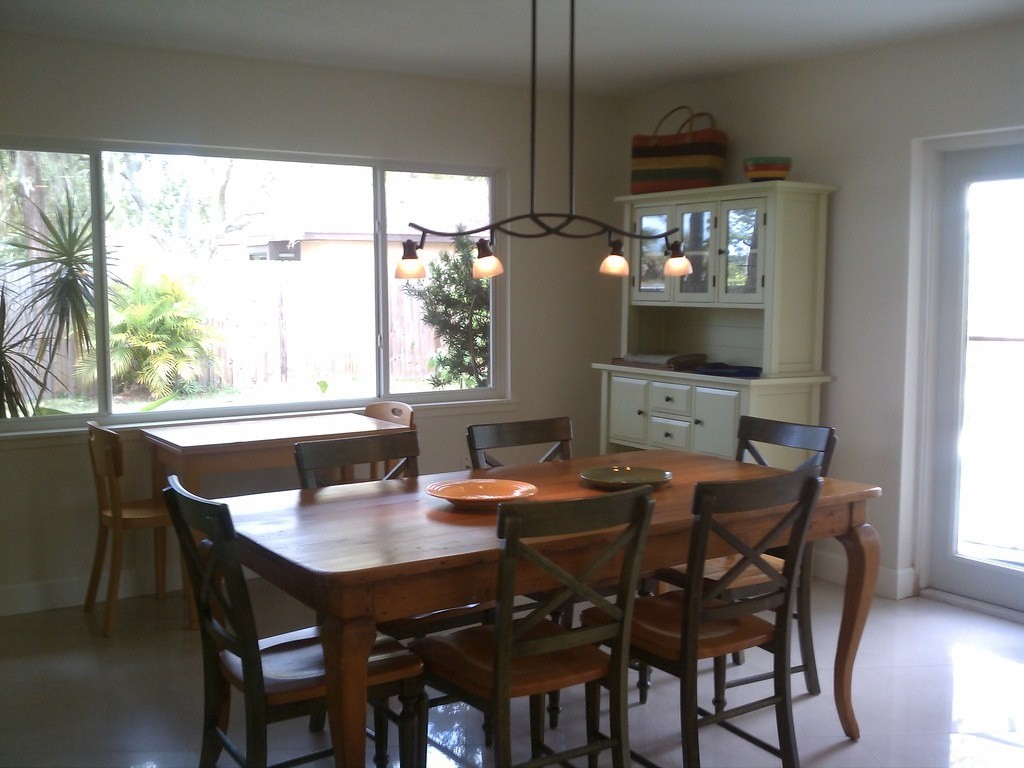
[427,478,537,510]
[744,157,791,182]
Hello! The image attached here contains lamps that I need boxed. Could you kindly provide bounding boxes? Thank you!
[392,0,694,282]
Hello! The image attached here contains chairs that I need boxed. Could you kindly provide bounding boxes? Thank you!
[81,398,839,768]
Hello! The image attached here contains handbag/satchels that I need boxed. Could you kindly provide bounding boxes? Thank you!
[630,109,725,190]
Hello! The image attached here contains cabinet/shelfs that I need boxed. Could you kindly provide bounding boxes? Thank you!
[591,178,839,469]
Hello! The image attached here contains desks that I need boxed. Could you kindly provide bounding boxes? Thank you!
[202,445,883,768]
[140,410,415,631]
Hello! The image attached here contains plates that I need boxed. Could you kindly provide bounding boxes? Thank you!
[578,466,673,491]
